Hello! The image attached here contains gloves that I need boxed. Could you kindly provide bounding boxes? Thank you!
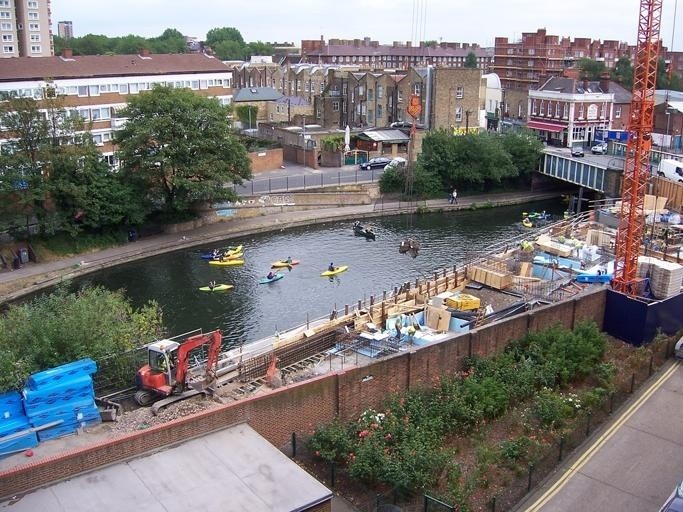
[201,244,246,265]
[257,273,285,284]
[353,225,375,241]
[562,195,578,201]
[199,283,234,291]
[320,265,348,276]
[273,258,300,266]
[522,211,552,228]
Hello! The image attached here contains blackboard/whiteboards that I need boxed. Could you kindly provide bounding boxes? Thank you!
[133,327,222,417]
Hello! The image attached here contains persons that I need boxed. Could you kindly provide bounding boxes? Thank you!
[524,208,547,228]
[394,313,416,348]
[644,238,666,252]
[208,280,216,289]
[597,268,608,275]
[448,185,459,205]
[282,256,292,263]
[266,271,274,279]
[213,248,228,263]
[519,239,535,254]
[328,262,336,271]
[557,231,592,270]
[353,219,375,233]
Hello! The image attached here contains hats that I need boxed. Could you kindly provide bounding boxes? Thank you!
[571,147,584,157]
[591,143,608,155]
[360,157,392,170]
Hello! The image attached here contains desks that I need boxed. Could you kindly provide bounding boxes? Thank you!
[665,112,670,149]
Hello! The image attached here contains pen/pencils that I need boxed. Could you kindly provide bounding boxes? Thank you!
[657,158,683,183]
